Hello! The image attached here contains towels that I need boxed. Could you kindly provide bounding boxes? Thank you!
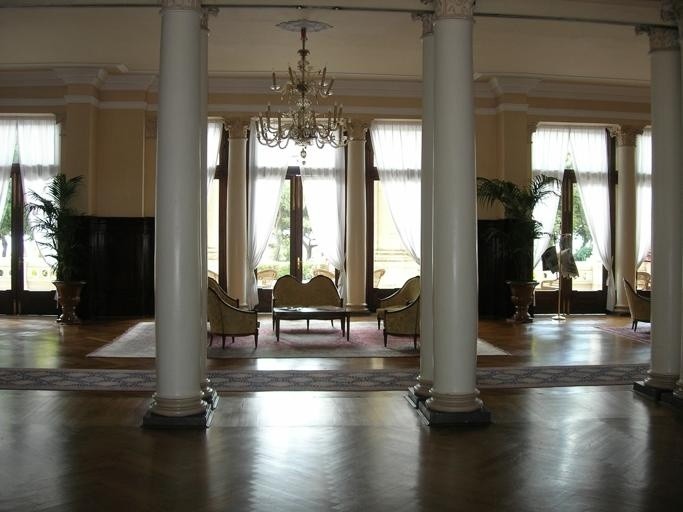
[541,246,579,280]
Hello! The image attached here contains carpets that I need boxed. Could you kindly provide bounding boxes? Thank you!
[85,320,511,358]
[0,363,650,392]
[592,325,650,345]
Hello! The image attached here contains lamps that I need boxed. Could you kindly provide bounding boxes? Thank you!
[254,18,353,168]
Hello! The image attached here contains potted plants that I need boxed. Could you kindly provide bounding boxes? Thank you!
[478,173,562,323]
[28,172,89,326]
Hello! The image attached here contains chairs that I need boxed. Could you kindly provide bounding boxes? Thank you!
[207,275,422,350]
[207,270,385,288]
[622,270,651,332]
